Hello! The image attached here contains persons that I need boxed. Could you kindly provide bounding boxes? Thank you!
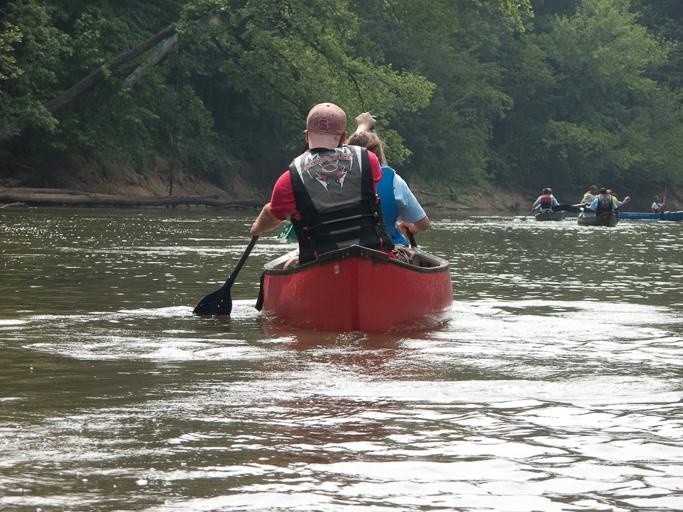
[341,111,429,247]
[249,102,430,271]
[531,184,663,218]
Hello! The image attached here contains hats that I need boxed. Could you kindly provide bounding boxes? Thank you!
[303,100,348,148]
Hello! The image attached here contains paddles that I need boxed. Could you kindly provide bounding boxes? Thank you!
[193,236,257,317]
[660,187,668,220]
[552,204,590,211]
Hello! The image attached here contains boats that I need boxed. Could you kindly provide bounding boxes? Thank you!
[256,243,454,335]
[534,210,566,221]
[577,209,619,227]
[618,210,683,221]
